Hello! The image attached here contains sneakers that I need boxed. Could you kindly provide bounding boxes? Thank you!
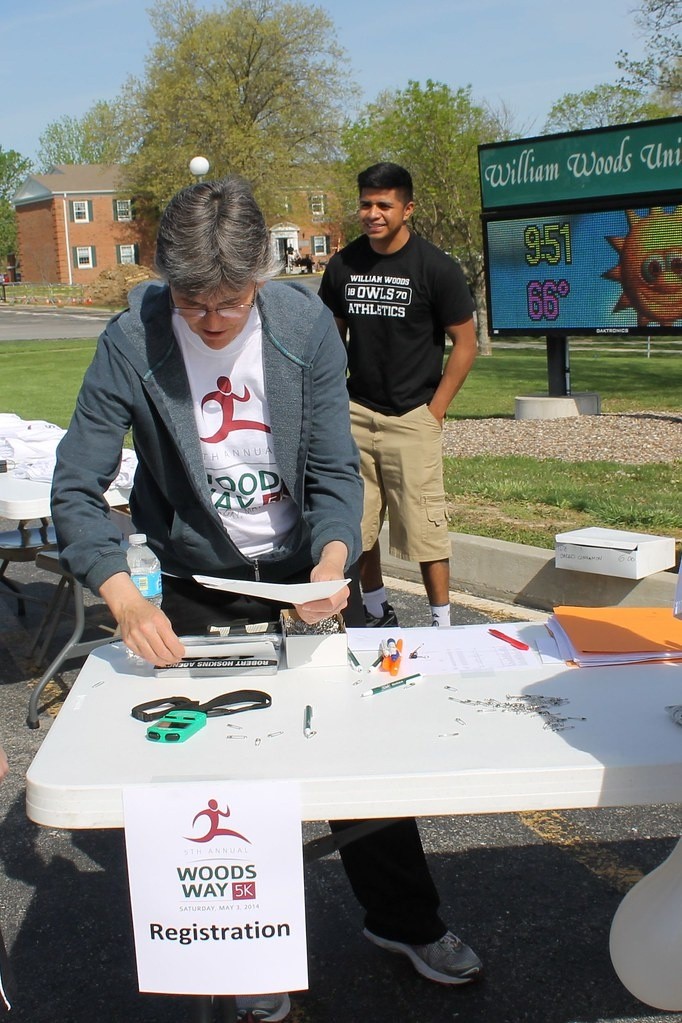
[361,923,482,986]
[234,994,291,1022]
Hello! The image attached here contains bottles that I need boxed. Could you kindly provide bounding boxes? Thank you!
[124,533,163,663]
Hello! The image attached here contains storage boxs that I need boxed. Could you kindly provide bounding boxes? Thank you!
[153,635,283,678]
[554,527,676,580]
[108,505,136,549]
[280,608,349,669]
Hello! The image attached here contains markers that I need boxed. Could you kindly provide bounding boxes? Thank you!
[388,638,403,676]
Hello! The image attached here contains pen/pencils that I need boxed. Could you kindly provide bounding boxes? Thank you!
[305,705,312,738]
[368,655,383,672]
[347,647,362,673]
[361,673,421,696]
[489,629,529,650]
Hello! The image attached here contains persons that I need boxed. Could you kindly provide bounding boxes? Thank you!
[318,162,476,628]
[50,182,481,1022]
[287,244,311,271]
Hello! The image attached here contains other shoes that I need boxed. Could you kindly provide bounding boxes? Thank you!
[363,601,398,627]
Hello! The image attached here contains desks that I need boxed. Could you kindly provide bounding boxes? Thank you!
[0,438,134,670]
[26,622,682,1022]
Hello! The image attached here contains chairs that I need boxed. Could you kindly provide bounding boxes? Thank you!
[0,517,134,729]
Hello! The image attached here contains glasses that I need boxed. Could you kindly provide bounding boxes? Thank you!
[170,277,257,318]
